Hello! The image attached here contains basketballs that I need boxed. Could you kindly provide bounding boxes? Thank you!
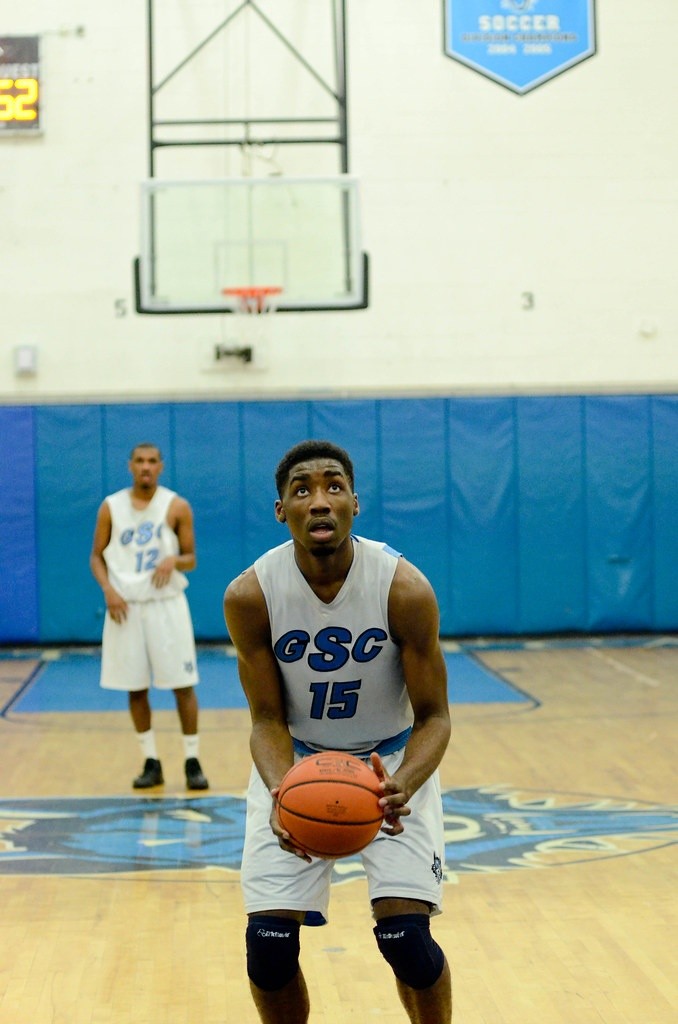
[275,751,386,859]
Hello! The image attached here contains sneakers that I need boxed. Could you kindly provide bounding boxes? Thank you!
[185,758,208,789]
[132,758,163,788]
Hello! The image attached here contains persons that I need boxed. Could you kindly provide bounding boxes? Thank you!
[223,440,451,1024]
[89,444,209,792]
